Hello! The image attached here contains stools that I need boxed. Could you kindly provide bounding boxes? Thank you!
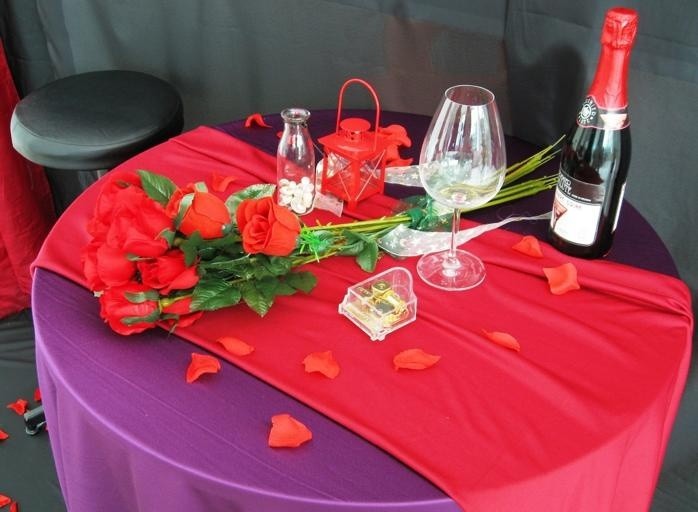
[9,69,179,180]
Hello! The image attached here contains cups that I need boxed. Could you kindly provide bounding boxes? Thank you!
[415,84,507,293]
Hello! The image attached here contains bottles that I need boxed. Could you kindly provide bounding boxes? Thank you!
[546,6,639,262]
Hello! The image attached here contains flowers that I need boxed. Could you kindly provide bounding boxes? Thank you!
[90,133,570,339]
